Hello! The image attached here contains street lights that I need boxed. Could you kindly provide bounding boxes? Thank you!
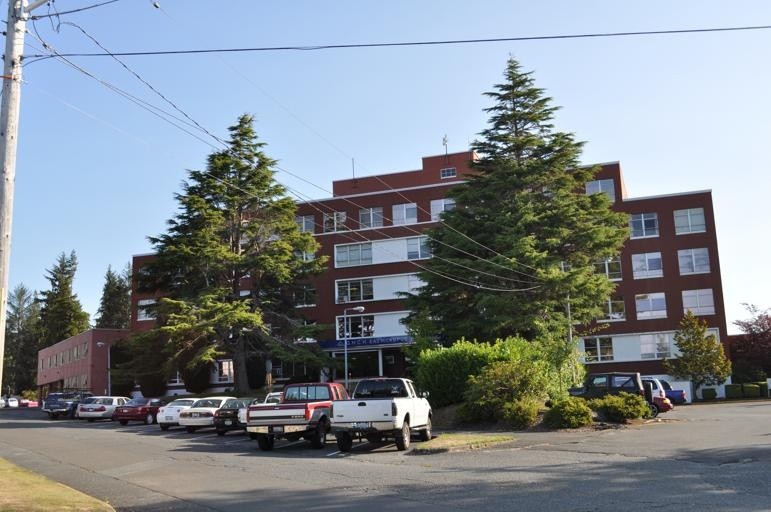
[95,341,111,396]
[343,305,365,389]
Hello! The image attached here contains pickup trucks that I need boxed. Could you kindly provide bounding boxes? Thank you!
[329,374,436,453]
[237,390,312,428]
[244,380,352,454]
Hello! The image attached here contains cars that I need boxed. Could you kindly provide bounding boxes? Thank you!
[213,397,264,437]
[659,378,687,406]
[7,398,19,408]
[154,397,201,433]
[76,396,121,417]
[640,394,676,420]
[78,395,133,422]
[176,396,238,433]
[0,400,6,408]
[111,397,169,427]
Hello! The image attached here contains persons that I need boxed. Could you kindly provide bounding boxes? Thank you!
[133,385,144,399]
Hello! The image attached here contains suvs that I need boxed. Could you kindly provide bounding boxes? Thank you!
[564,370,653,405]
[42,391,95,420]
[608,374,666,398]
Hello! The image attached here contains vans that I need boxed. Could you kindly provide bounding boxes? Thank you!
[659,379,672,391]
